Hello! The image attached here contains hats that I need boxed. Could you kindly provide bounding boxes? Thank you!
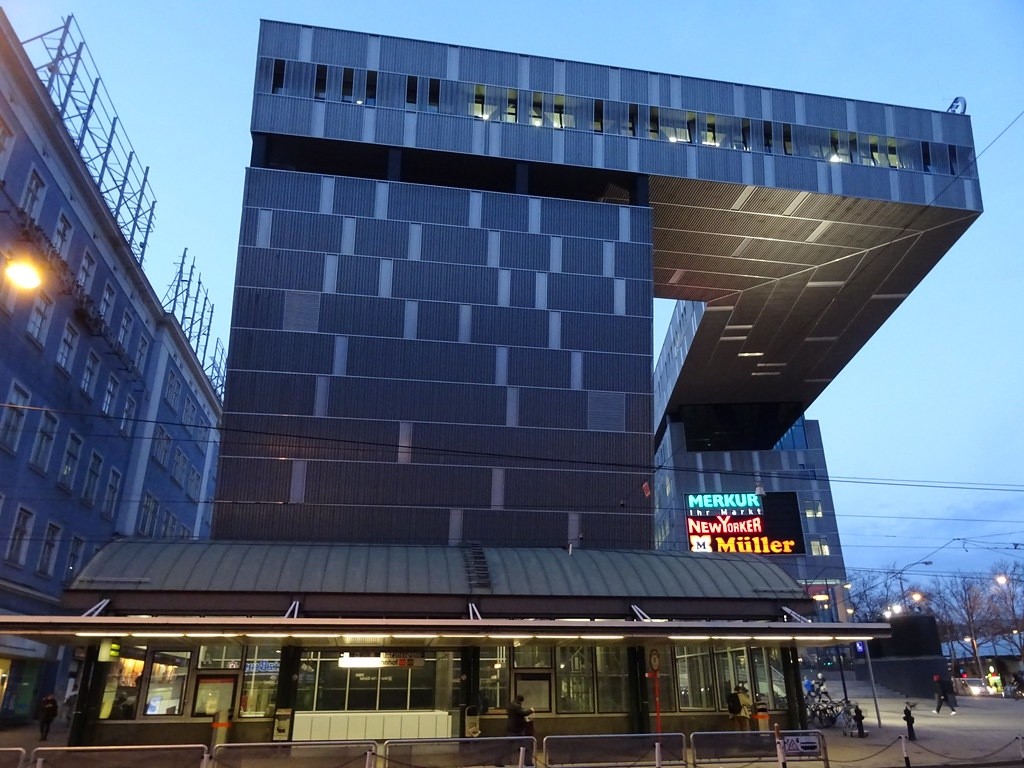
[515,694,524,702]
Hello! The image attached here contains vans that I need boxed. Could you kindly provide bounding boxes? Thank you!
[953,677,996,697]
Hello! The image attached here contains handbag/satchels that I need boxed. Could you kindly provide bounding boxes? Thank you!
[820,683,827,692]
[524,720,535,736]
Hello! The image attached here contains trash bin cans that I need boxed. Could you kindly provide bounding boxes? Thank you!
[209,712,233,756]
[753,711,770,746]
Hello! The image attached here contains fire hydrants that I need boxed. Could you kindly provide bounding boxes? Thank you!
[902,706,916,742]
[851,704,866,738]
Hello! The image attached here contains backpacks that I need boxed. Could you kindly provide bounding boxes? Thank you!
[726,693,742,714]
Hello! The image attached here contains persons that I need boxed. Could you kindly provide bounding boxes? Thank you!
[504,695,535,767]
[803,673,831,702]
[732,686,752,731]
[37,684,78,741]
[932,675,956,715]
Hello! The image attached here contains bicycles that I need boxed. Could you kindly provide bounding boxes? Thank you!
[803,690,855,729]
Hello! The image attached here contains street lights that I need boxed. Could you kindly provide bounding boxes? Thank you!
[899,561,934,614]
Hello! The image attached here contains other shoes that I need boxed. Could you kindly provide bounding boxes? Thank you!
[950,711,956,716]
[931,709,939,714]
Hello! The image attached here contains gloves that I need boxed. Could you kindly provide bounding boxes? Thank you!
[524,716,530,722]
[530,707,535,713]
